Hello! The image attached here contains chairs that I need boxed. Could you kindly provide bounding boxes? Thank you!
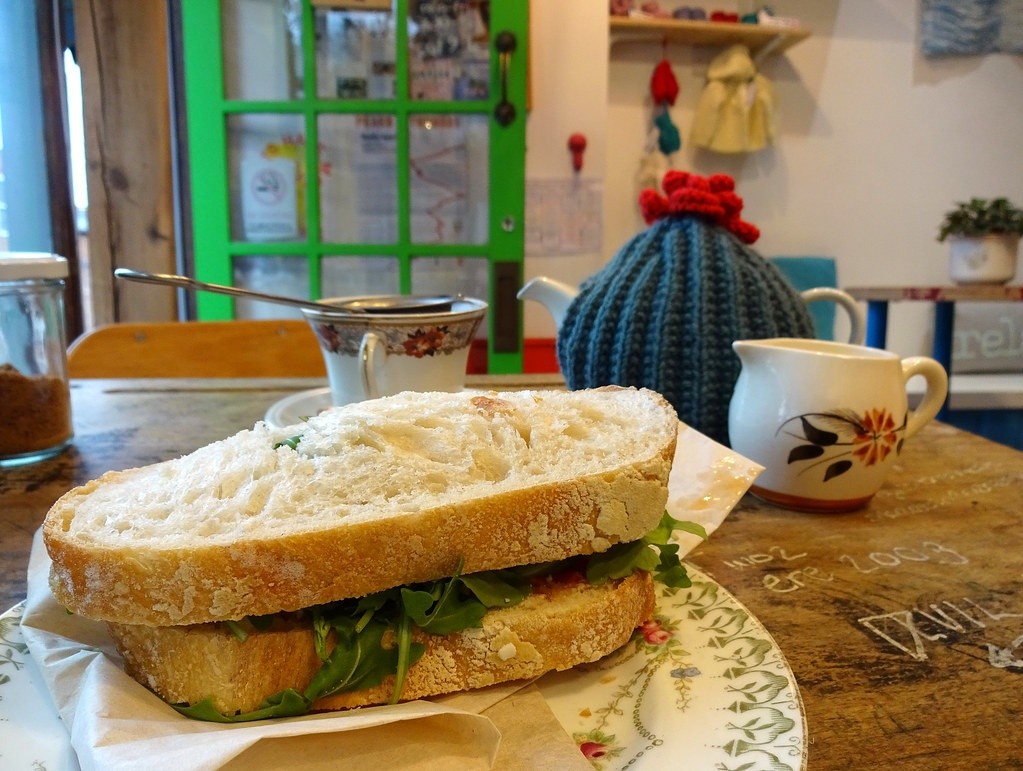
[50,318,327,377]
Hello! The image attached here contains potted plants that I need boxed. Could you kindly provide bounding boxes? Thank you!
[936,196,1023,282]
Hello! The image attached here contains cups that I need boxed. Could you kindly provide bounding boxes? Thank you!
[301,293,491,408]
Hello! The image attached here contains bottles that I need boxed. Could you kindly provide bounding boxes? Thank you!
[0,252,75,467]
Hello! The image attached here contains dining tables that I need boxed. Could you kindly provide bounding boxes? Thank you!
[0,374,1023,771]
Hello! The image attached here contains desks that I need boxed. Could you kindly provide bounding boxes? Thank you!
[843,286,1023,423]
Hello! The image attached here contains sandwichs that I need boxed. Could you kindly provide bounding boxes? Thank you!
[45,384,678,723]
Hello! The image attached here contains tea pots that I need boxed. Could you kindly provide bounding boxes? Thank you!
[728,332,951,515]
[514,169,866,459]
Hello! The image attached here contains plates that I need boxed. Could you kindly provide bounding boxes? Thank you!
[263,385,336,435]
[0,554,810,771]
